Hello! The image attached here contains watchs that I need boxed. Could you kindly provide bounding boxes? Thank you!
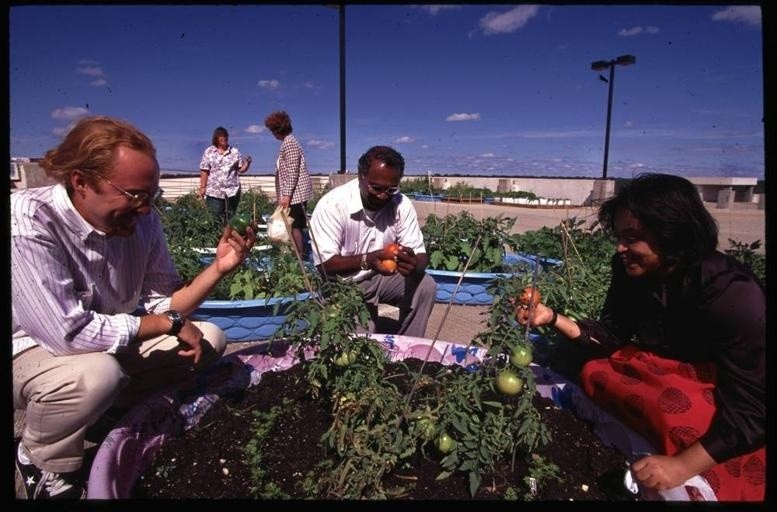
[164,310,186,336]
[360,252,373,270]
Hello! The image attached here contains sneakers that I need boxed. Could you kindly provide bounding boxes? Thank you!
[14,453,87,501]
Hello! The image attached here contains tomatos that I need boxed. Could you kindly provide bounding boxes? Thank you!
[329,242,540,453]
[231,212,252,236]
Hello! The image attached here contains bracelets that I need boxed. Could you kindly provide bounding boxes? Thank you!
[200,186,206,189]
[547,308,557,328]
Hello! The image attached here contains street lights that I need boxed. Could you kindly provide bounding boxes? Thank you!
[590,54,637,179]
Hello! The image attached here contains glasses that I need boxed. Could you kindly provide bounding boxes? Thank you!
[81,168,164,208]
[362,175,401,196]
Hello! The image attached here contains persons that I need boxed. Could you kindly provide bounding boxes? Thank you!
[11,115,260,500]
[507,172,766,500]
[198,126,252,226]
[264,110,312,262]
[308,146,438,338]
